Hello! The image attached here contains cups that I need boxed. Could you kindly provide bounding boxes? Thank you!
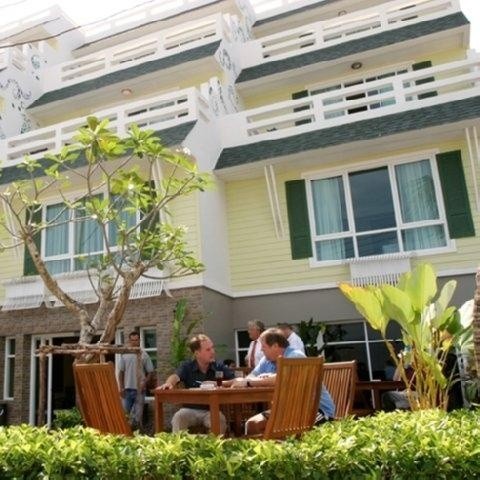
[215,371,223,389]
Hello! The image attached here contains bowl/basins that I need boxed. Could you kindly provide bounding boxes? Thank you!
[221,380,234,388]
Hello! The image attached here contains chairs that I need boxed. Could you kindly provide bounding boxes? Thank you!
[74,361,134,434]
[264,357,358,439]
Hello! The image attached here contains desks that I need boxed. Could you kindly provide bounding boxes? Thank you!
[355,380,406,415]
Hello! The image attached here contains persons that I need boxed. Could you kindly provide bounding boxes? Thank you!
[277,322,306,353]
[245,320,267,368]
[117,331,155,428]
[222,359,237,371]
[156,332,236,439]
[226,326,336,437]
